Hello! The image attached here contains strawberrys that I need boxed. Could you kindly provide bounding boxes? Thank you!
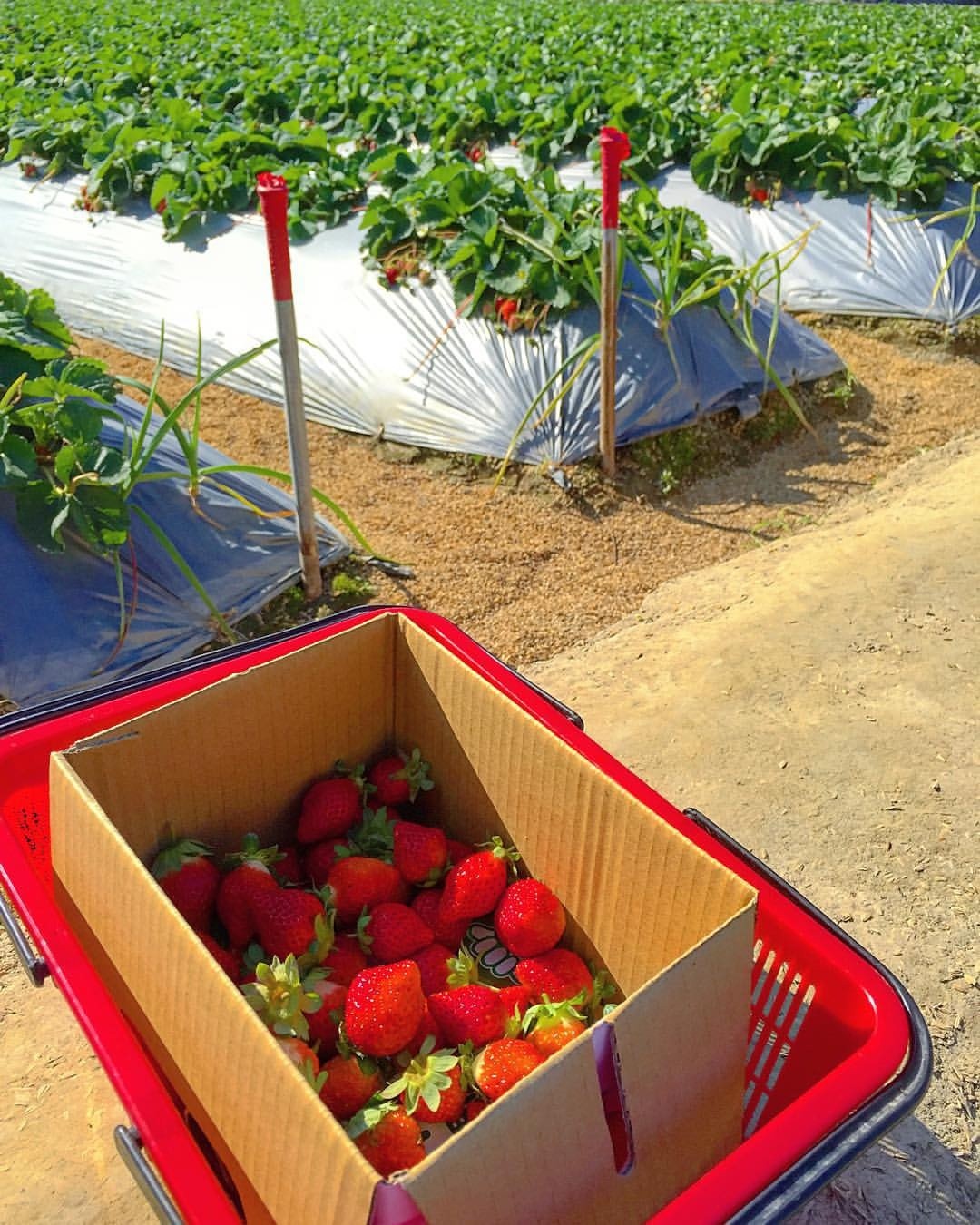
[7,111,776,340]
[122,738,590,1191]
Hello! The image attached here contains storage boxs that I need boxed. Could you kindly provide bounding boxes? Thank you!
[52,611,759,1225]
